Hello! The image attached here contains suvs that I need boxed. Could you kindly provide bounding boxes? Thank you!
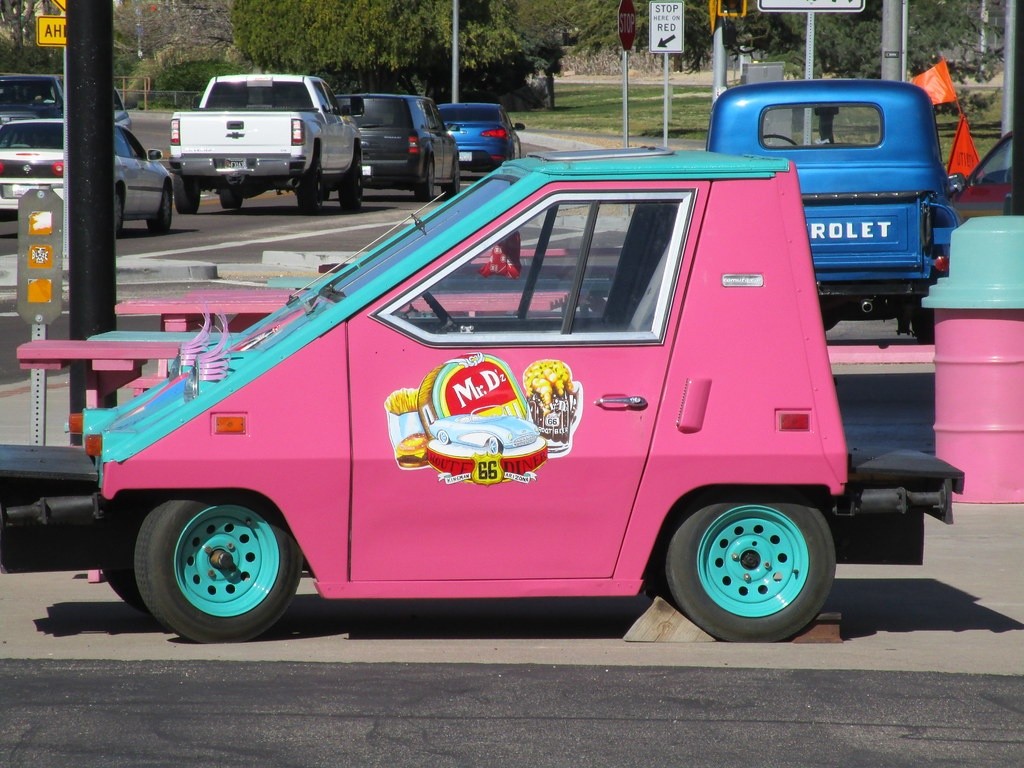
[331,94,462,203]
[706,77,962,344]
[0,71,132,132]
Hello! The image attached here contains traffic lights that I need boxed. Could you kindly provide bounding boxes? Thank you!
[717,0,747,19]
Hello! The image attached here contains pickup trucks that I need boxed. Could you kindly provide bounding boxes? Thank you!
[167,75,365,218]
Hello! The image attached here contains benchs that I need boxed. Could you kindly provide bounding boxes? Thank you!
[602,204,677,332]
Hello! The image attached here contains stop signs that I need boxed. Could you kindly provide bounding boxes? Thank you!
[616,1,638,53]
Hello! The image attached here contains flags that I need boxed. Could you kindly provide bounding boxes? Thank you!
[947,114,985,179]
[912,55,957,105]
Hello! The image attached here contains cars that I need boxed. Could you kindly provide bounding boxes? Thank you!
[0,116,176,240]
[436,103,526,173]
[94,149,854,646]
[949,129,1014,223]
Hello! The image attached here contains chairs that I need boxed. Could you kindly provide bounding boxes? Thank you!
[281,84,313,108]
[219,86,249,108]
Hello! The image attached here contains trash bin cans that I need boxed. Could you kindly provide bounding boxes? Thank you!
[920,214,1024,504]
